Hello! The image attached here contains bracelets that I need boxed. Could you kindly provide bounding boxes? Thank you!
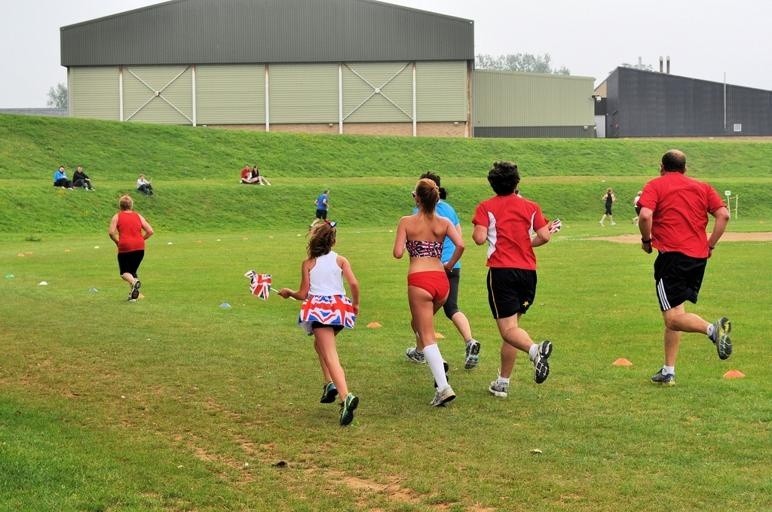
[641,237,651,243]
[707,245,714,249]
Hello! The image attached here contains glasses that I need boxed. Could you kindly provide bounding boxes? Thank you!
[412,190,416,199]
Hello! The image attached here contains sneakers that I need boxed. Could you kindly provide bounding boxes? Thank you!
[651,370,676,387]
[488,372,508,395]
[534,341,552,384]
[131,280,141,298]
[320,384,337,403]
[709,317,732,360]
[465,340,480,370]
[340,393,359,425]
[406,340,455,406]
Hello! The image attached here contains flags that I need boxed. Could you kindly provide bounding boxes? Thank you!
[549,217,561,234]
[243,270,273,301]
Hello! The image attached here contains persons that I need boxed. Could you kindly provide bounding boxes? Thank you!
[471,159,554,397]
[631,190,643,226]
[635,149,732,384]
[52,165,73,190]
[275,220,359,426]
[405,171,480,371]
[393,178,466,406]
[251,165,271,186]
[240,165,255,183]
[135,173,153,196]
[71,166,95,192]
[309,189,331,229]
[108,194,154,301]
[599,187,617,227]
